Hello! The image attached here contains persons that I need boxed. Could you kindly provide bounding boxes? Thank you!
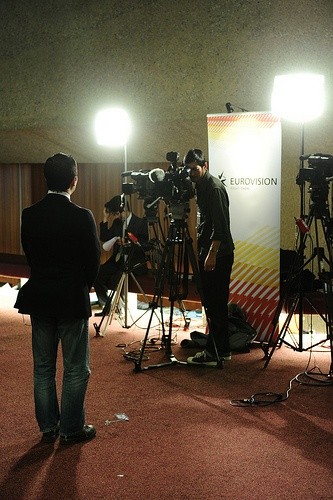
[93,195,149,316]
[184,148,235,366]
[14,153,101,445]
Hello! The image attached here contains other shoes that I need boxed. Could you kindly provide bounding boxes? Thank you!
[95,309,105,316]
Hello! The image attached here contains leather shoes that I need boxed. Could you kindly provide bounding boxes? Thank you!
[60,424,96,444]
[41,425,60,443]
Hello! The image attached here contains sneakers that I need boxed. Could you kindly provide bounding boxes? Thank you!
[187,351,217,365]
[221,352,231,360]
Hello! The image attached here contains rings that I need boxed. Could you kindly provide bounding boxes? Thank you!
[212,269,213,270]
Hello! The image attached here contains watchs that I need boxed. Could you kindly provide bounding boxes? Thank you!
[210,247,218,252]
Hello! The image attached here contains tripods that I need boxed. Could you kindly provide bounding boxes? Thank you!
[97,200,223,371]
[260,184,333,376]
